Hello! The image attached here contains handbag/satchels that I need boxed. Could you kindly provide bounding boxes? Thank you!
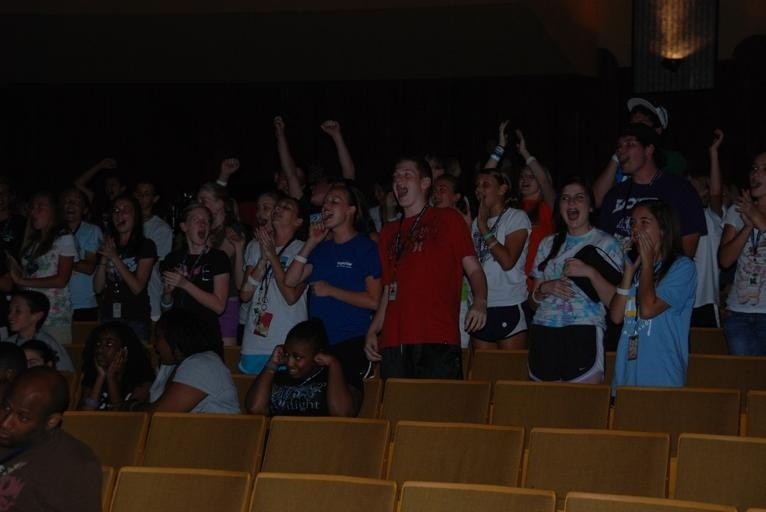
[568,244,623,305]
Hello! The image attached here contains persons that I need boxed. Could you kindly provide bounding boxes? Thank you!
[528,181,622,383]
[590,97,735,328]
[95,158,245,350]
[240,109,474,418]
[466,169,532,350]
[3,366,105,512]
[609,199,697,426]
[133,311,240,411]
[721,148,765,356]
[483,119,557,289]
[2,158,124,375]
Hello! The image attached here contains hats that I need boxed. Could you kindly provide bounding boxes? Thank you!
[627,98,669,130]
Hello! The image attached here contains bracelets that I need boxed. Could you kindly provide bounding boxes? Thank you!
[490,146,503,161]
[483,233,500,248]
[531,288,542,305]
[525,155,535,166]
[615,289,630,297]
